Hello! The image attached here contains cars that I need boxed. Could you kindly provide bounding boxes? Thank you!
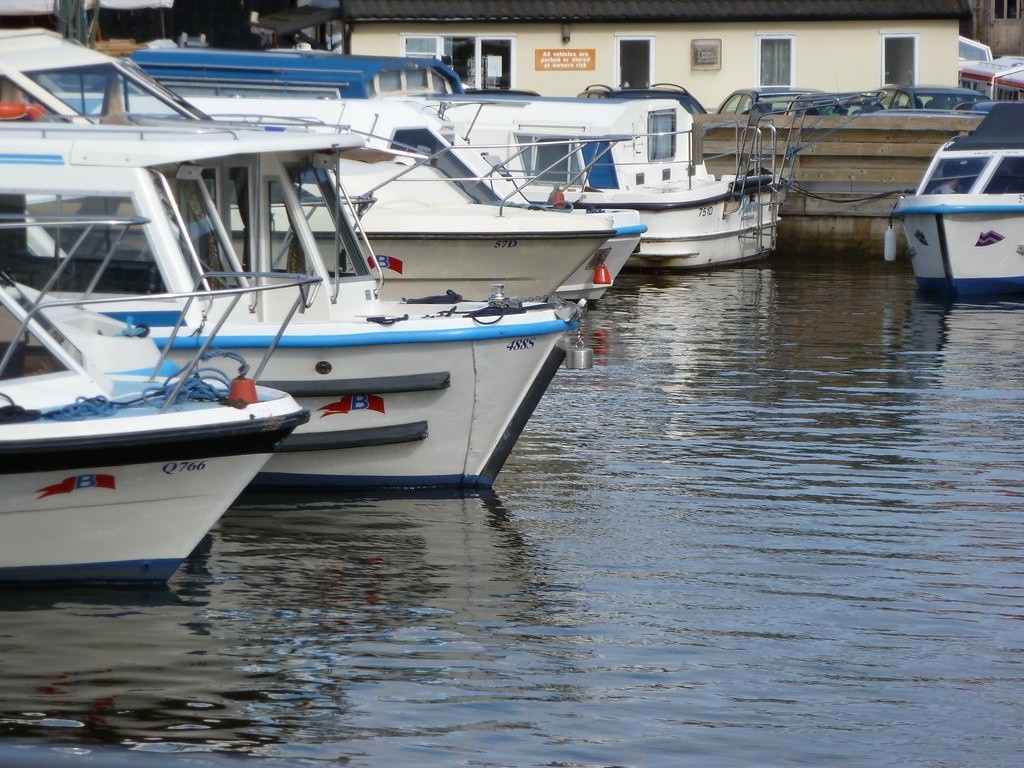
[713,87,848,115]
[784,93,885,116]
[868,84,991,113]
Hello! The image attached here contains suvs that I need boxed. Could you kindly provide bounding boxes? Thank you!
[576,83,708,114]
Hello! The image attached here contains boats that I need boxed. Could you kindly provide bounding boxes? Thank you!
[0,214,324,586]
[892,102,1024,297]
[0,121,593,493]
[0,28,889,308]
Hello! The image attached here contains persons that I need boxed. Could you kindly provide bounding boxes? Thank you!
[930,159,994,194]
